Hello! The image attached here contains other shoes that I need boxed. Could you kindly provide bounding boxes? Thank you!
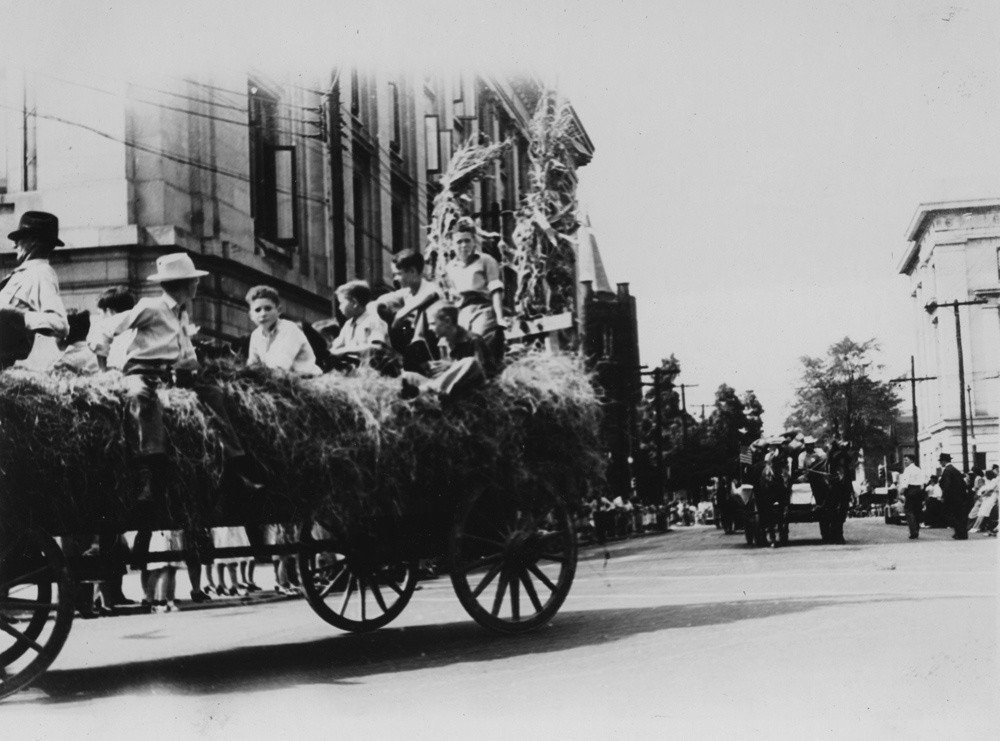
[84,572,334,614]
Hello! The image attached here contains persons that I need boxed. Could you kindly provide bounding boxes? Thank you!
[666,497,712,527]
[777,425,828,483]
[0,210,210,377]
[574,490,663,540]
[244,219,511,395]
[899,454,923,539]
[60,521,335,620]
[937,453,968,540]
[889,463,1000,537]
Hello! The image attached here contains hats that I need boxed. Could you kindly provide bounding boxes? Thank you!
[147,253,209,281]
[938,453,952,461]
[8,211,65,246]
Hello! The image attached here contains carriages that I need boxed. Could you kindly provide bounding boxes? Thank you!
[733,440,862,548]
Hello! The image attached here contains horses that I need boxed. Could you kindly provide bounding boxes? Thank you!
[753,444,790,548]
[809,439,855,544]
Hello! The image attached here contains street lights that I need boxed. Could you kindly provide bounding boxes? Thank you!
[633,368,680,483]
[668,383,699,443]
[889,356,937,467]
[924,296,989,474]
